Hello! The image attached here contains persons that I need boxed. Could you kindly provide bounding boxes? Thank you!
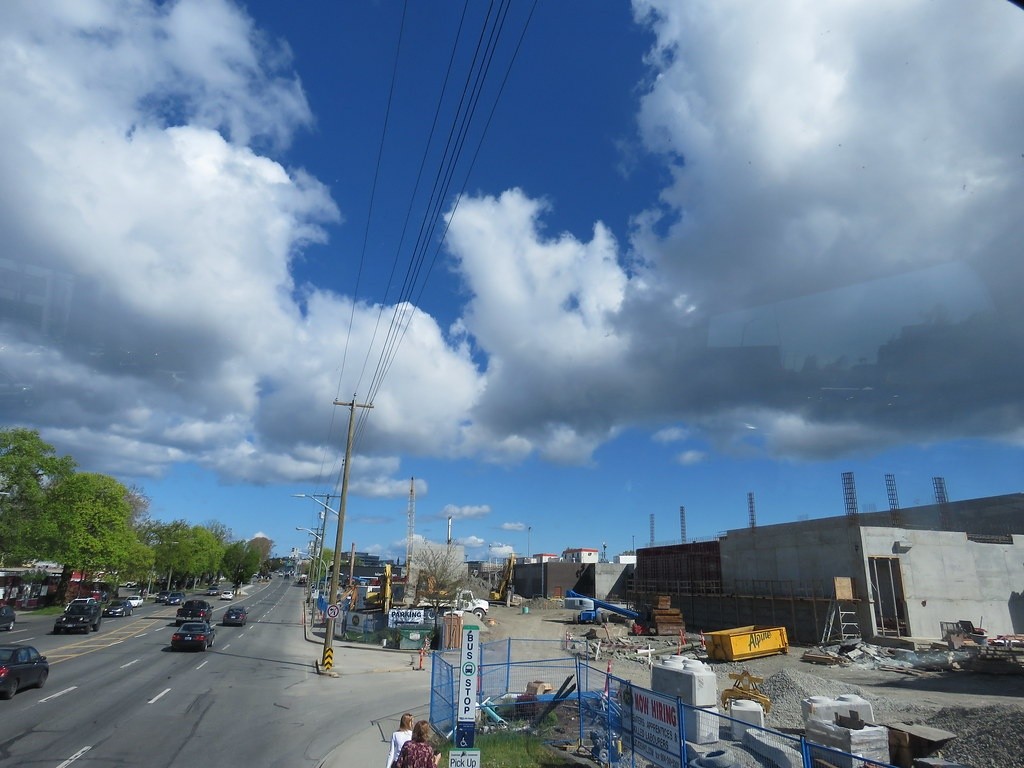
[386,713,414,768]
[393,720,441,768]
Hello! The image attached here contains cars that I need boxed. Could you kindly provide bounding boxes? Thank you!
[222,606,249,627]
[0,606,17,631]
[102,599,133,617]
[221,591,234,600]
[0,645,50,699]
[175,599,215,625]
[125,595,144,607]
[166,592,187,606]
[207,583,222,596]
[252,570,296,582]
[118,580,136,588]
[156,590,173,603]
[171,622,216,651]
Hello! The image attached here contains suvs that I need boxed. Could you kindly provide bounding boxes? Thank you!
[64,598,97,613]
[54,602,103,634]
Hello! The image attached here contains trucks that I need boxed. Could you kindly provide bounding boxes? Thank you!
[403,587,490,621]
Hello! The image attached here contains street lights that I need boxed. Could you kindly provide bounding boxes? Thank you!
[297,551,329,602]
[289,493,344,670]
[295,526,324,628]
